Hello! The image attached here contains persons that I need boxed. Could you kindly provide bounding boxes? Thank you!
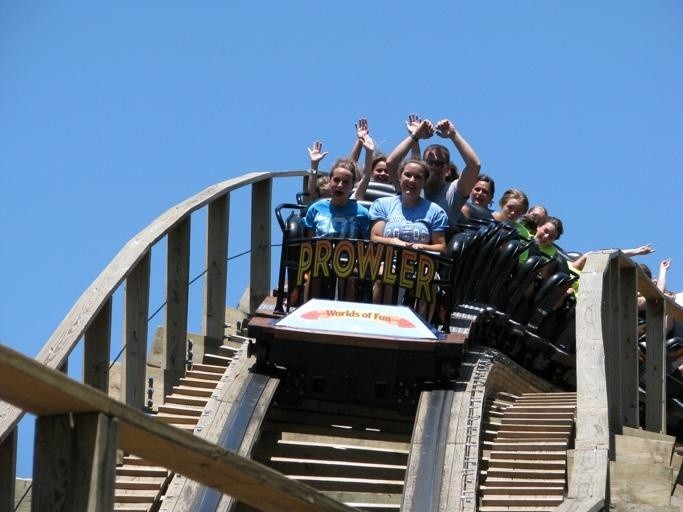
[286,112,683,374]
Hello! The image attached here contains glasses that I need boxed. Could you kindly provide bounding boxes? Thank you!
[425,158,446,166]
